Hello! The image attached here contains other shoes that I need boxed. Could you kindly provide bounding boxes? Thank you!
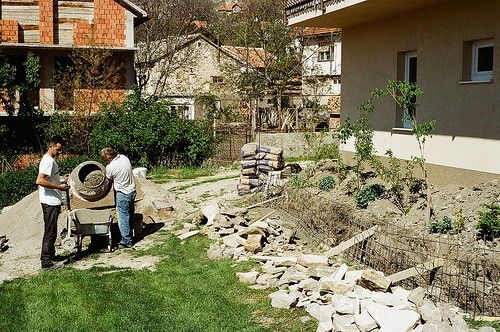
[54,255,69,264]
[111,243,132,248]
[41,261,57,268]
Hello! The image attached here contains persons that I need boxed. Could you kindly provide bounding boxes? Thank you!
[35,135,72,270]
[99,147,137,249]
